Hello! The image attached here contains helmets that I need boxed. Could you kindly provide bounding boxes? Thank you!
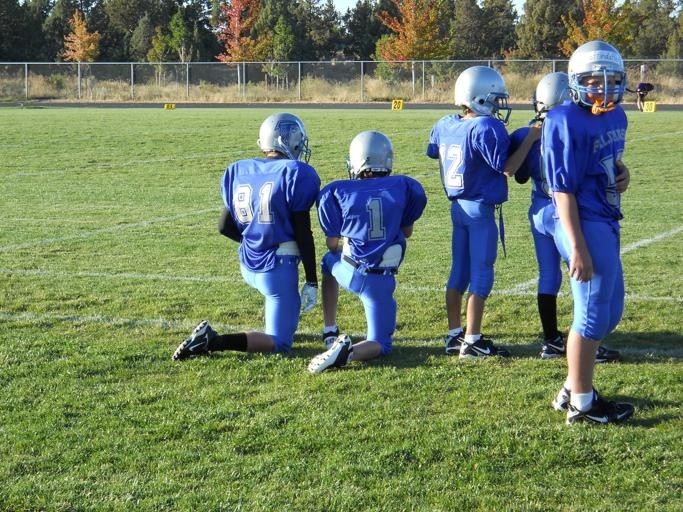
[258,112,311,164]
[346,131,393,178]
[533,72,570,120]
[568,40,627,109]
[454,65,512,126]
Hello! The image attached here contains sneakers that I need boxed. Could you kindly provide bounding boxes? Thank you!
[540,337,567,358]
[308,333,354,375]
[459,337,510,363]
[565,389,634,426]
[171,320,216,360]
[594,346,622,364]
[551,386,571,411]
[445,327,467,354]
[322,327,339,349]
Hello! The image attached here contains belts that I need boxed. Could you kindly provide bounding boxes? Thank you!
[343,257,398,275]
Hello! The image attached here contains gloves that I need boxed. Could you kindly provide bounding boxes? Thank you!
[301,285,318,312]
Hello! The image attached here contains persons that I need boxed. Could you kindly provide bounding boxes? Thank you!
[541,40,635,425]
[308,130,427,375]
[427,66,542,359]
[510,72,621,363]
[173,113,320,359]
